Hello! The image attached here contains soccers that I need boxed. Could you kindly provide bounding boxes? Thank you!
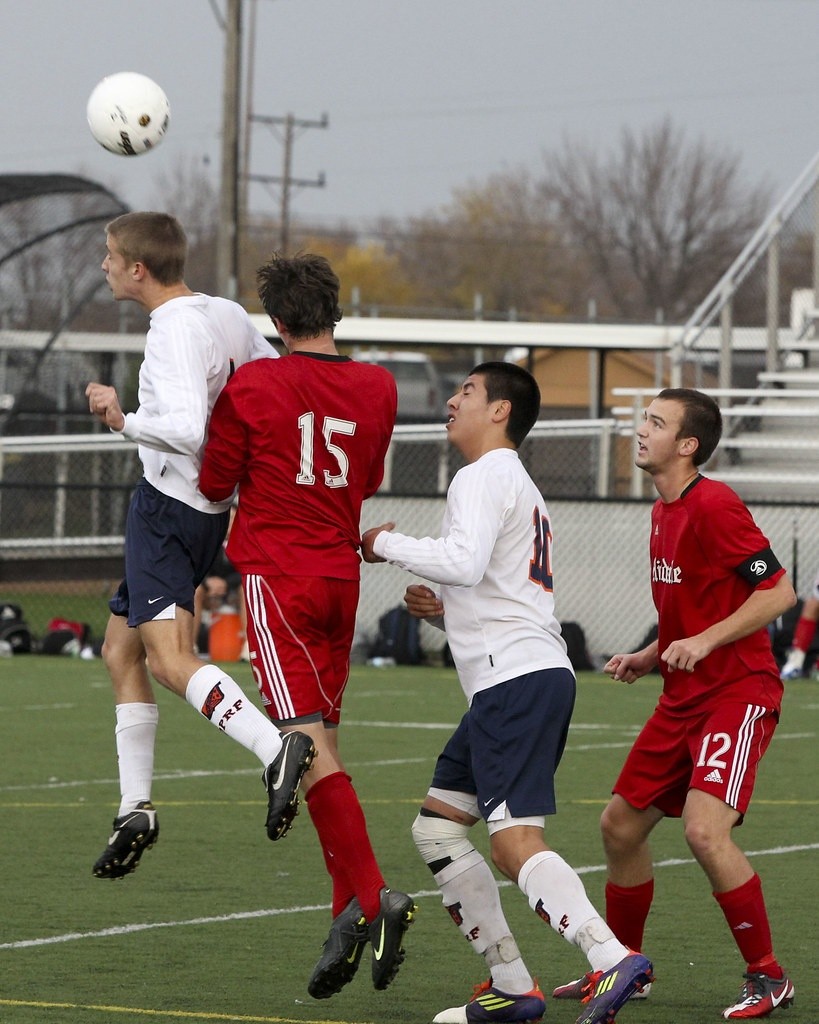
[86,72,171,157]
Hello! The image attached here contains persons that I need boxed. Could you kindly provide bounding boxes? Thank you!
[552,388,800,1017]
[780,580,819,679]
[355,360,654,1023]
[196,255,419,1001]
[83,210,316,886]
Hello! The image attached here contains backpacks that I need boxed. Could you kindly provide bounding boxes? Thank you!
[366,605,427,666]
[562,621,597,671]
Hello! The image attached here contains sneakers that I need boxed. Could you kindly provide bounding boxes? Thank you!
[552,970,653,1002]
[92,801,159,882]
[261,731,319,841]
[308,897,370,1000]
[574,945,656,1024]
[366,885,419,992]
[721,965,794,1020]
[432,976,546,1024]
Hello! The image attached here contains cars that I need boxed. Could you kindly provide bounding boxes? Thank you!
[348,347,443,419]
